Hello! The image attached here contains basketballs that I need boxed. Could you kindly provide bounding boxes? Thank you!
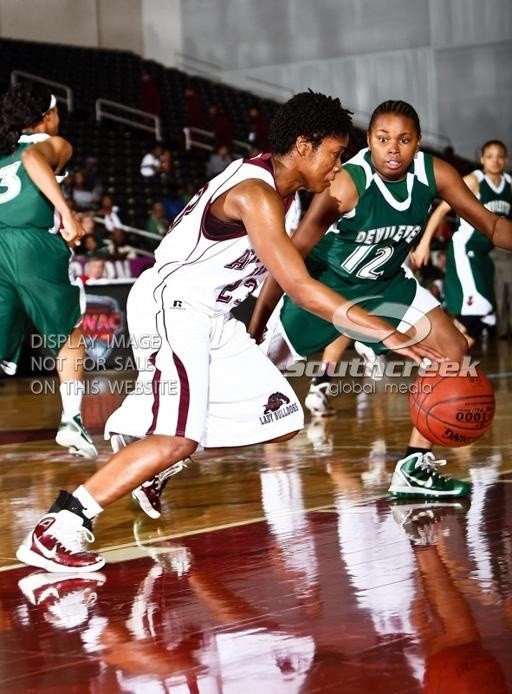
[407,359,495,447]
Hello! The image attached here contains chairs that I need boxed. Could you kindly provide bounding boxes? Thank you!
[298,190,314,221]
[0,36,185,278]
[185,73,283,207]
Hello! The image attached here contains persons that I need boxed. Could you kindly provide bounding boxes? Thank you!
[16,420,512,692]
[245,99,512,499]
[14,86,450,577]
[413,138,512,371]
[1,80,102,462]
[302,330,393,419]
[55,142,261,284]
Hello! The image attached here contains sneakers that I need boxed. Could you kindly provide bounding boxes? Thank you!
[16,376,472,576]
